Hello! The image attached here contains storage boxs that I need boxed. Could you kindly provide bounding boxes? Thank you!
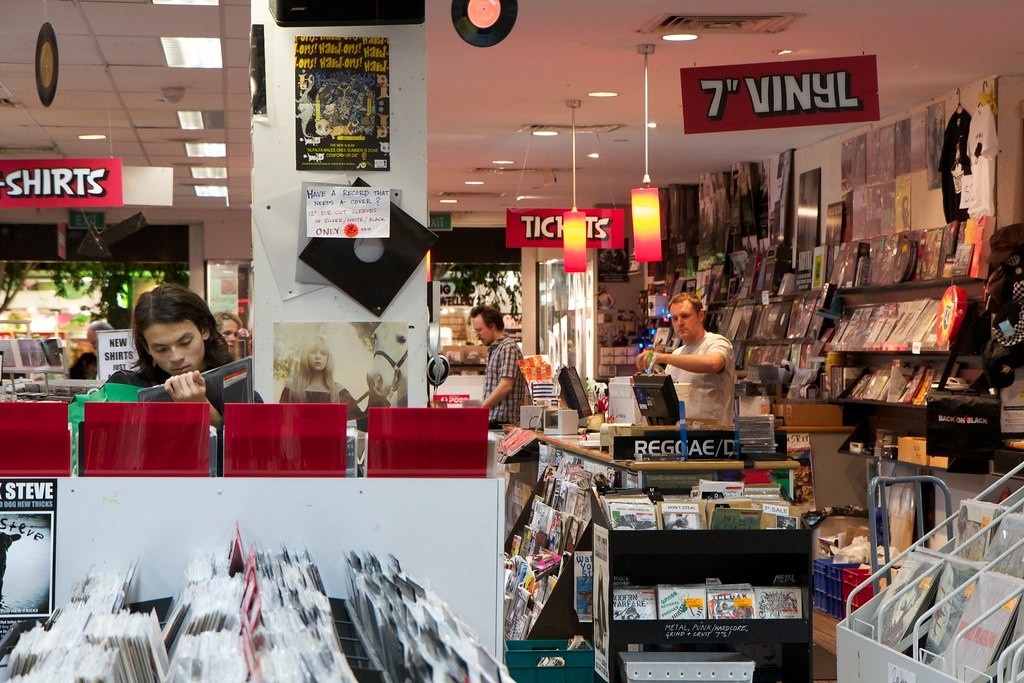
[619,651,757,683]
[503,639,595,683]
[841,568,874,619]
[898,435,929,466]
[813,557,871,621]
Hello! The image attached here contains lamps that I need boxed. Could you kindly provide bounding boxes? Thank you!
[562,99,587,274]
[630,44,664,263]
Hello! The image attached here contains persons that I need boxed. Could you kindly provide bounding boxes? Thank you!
[278,335,365,421]
[467,305,525,431]
[101,285,265,477]
[69,320,116,381]
[211,310,242,359]
[635,293,735,426]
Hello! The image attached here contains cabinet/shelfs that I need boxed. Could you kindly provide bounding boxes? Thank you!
[593,517,815,683]
[829,276,990,475]
